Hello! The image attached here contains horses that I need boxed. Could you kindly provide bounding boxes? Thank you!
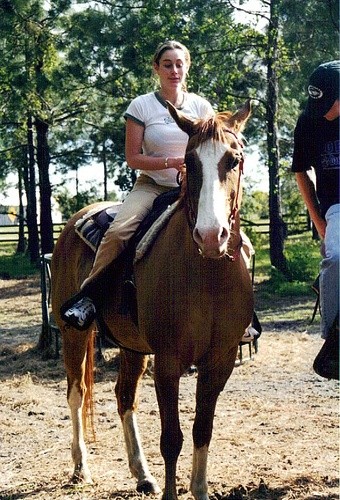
[50,98,256,500]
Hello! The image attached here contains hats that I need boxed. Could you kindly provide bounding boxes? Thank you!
[306,61,340,117]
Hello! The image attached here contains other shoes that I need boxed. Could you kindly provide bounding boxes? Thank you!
[324,345,339,378]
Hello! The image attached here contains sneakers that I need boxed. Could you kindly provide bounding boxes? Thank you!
[64,295,97,327]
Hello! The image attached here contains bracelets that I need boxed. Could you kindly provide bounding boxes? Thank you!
[165,156,168,168]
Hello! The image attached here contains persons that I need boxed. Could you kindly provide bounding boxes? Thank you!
[62,40,259,341]
[291,60,340,381]
